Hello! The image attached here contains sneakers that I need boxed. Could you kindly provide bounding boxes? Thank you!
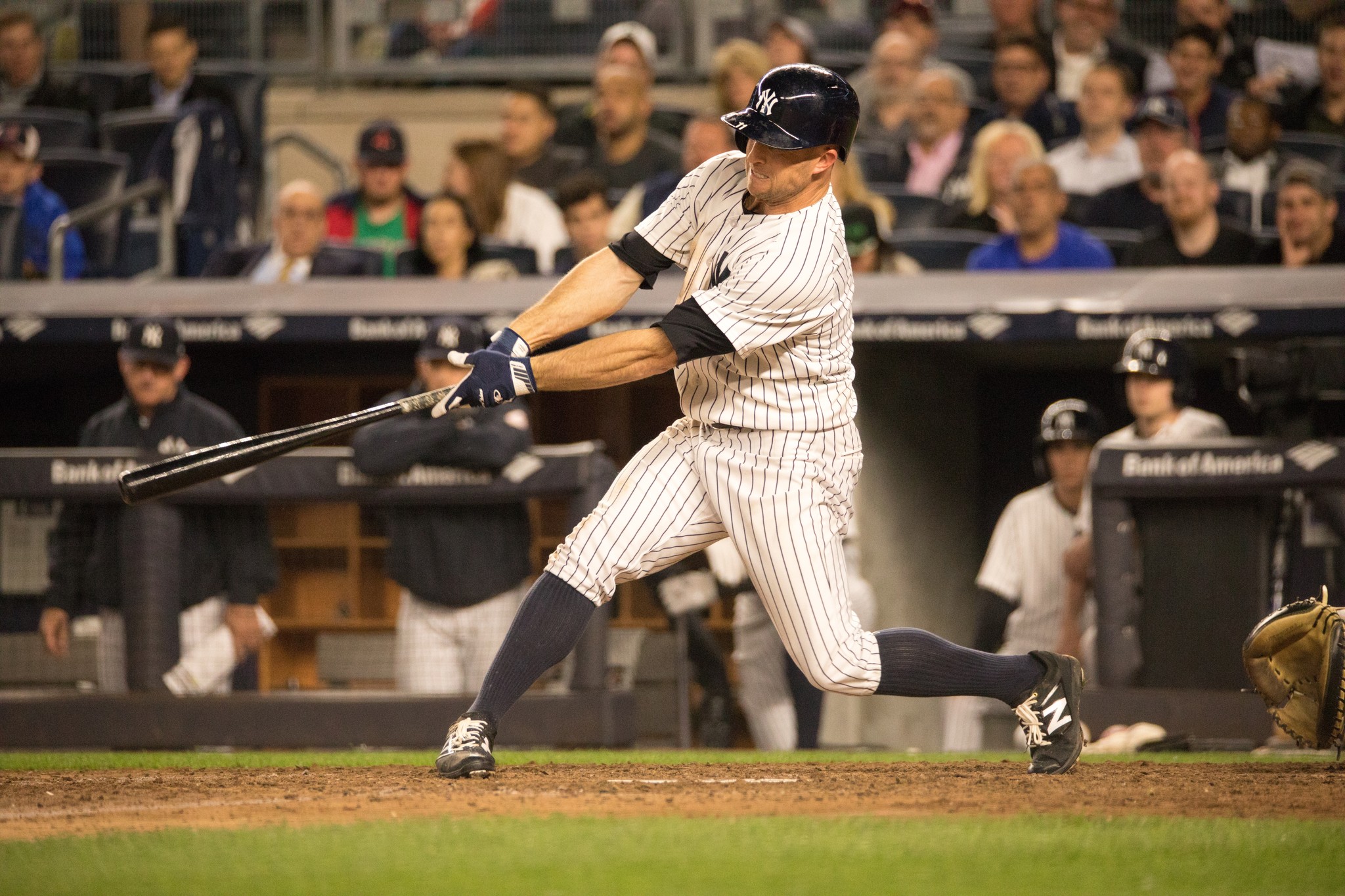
[1005,648,1086,775]
[436,712,497,776]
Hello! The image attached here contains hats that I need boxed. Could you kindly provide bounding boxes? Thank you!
[600,20,657,77]
[1125,91,1185,134]
[0,121,42,162]
[121,318,178,369]
[359,123,402,168]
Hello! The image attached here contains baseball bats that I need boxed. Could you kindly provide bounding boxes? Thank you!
[121,380,465,501]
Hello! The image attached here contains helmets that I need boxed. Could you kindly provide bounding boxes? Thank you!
[1116,324,1184,377]
[720,64,860,164]
[1034,397,1101,447]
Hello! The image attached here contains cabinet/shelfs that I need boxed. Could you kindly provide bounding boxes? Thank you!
[259,373,653,698]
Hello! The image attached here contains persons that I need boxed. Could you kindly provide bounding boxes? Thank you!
[429,62,1083,776]
[0,1,1345,751]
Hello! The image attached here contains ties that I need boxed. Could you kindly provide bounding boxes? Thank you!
[280,253,298,284]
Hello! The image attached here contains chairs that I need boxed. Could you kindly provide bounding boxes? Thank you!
[22,0,1345,284]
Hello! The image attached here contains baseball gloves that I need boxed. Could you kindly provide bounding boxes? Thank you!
[1241,586,1344,754]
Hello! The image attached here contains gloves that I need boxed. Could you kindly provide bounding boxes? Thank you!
[432,327,537,420]
